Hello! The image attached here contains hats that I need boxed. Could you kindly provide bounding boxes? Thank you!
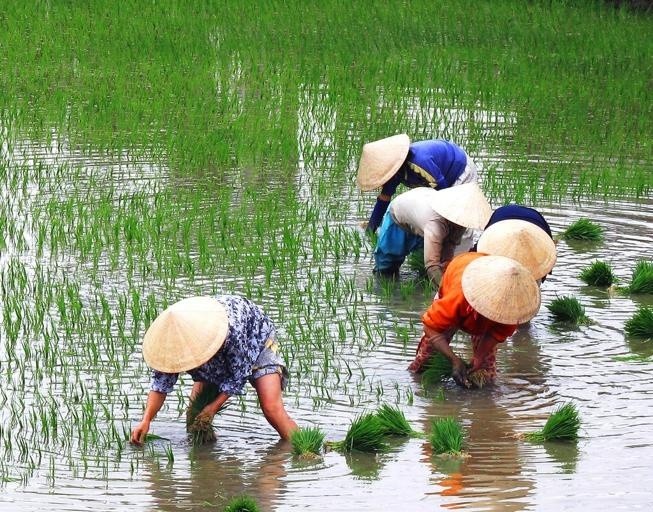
[357,134,410,191]
[429,182,493,229]
[462,256,541,326]
[142,296,228,374]
[477,219,557,280]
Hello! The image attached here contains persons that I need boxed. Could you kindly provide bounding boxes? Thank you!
[471,206,559,328]
[356,135,479,287]
[130,293,303,445]
[371,184,492,292]
[409,250,543,389]
